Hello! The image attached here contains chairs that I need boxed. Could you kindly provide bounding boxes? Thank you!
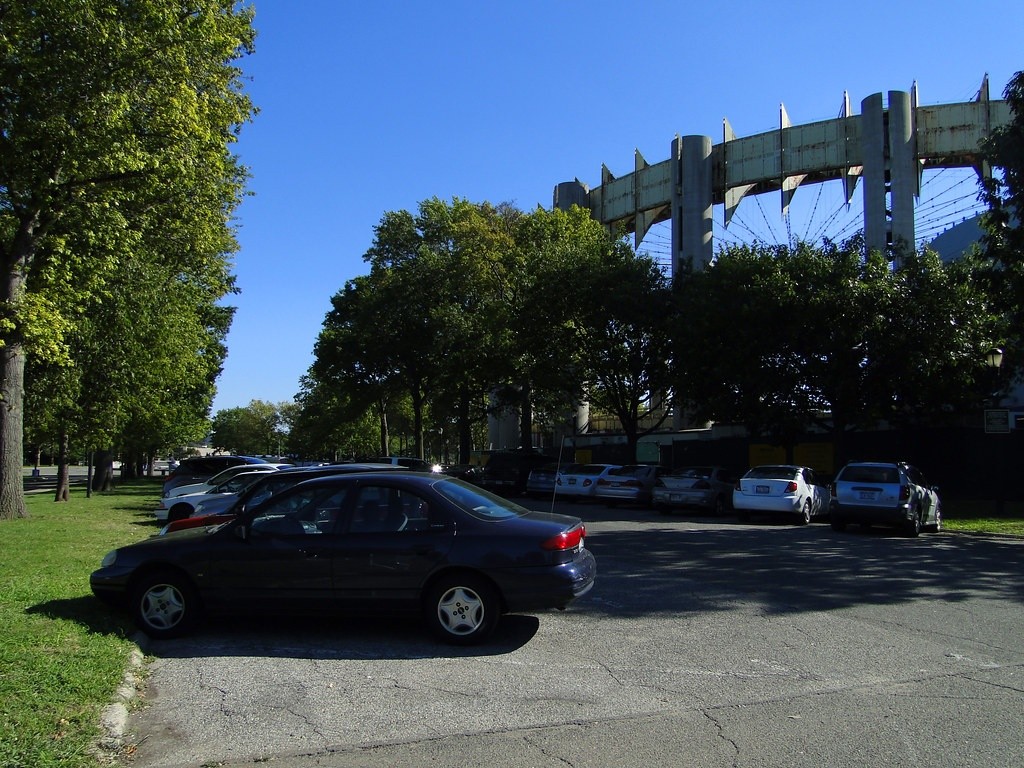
[384,497,408,532]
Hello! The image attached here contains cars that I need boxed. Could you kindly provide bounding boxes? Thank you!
[153,451,519,539]
[524,467,558,498]
[731,463,831,526]
[553,463,624,505]
[652,464,736,517]
[591,462,673,509]
[89,469,598,649]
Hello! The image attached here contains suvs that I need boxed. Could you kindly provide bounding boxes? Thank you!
[825,457,942,538]
